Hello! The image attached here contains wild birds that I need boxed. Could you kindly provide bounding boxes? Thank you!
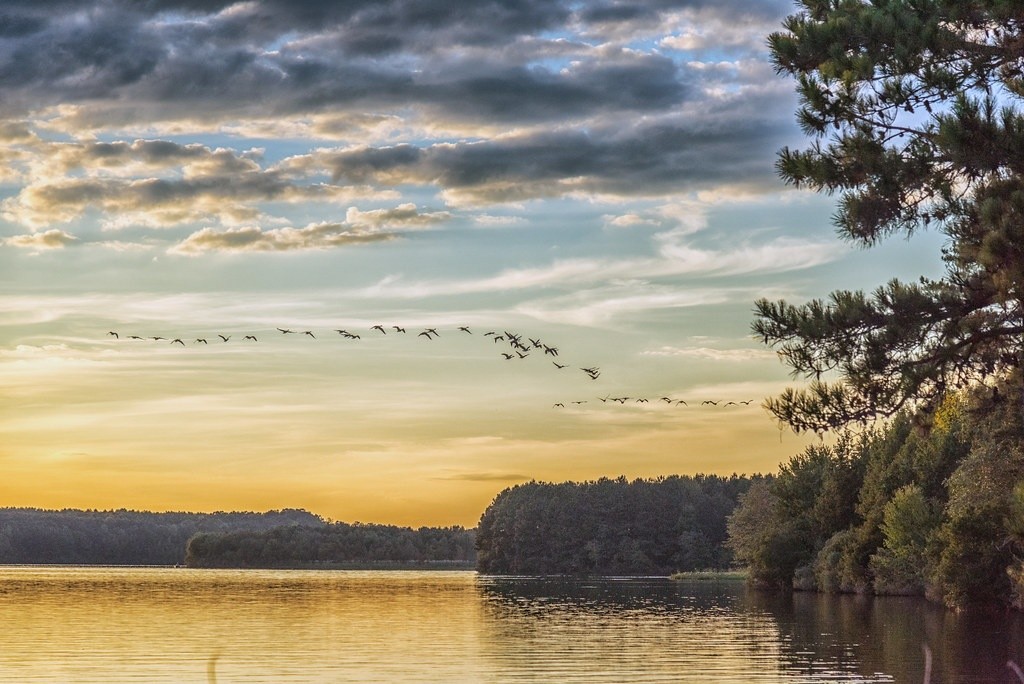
[242,336,258,341]
[107,331,119,339]
[128,336,143,340]
[458,326,471,334]
[485,329,568,370]
[171,339,185,346]
[148,336,164,341]
[418,328,440,340]
[218,335,231,343]
[194,339,207,345]
[300,330,316,340]
[334,329,360,341]
[370,325,386,335]
[549,393,754,411]
[579,365,600,381]
[277,327,296,334]
[393,326,405,333]
